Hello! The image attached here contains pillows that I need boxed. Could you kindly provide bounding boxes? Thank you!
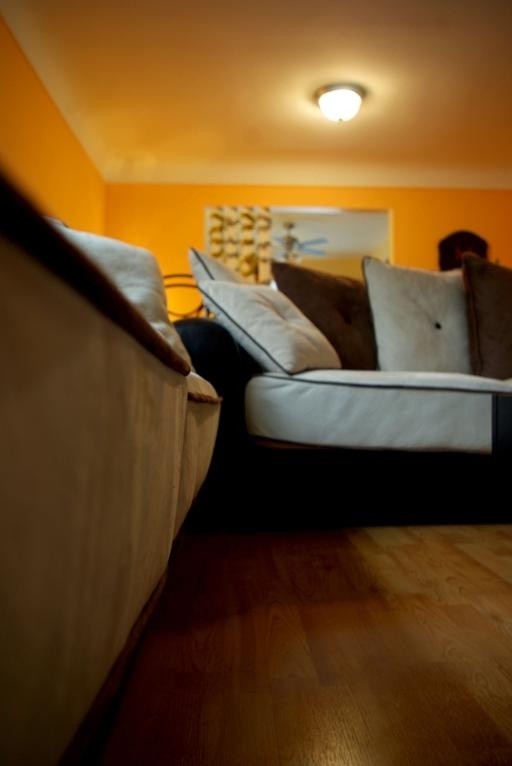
[269,258,374,371]
[364,255,473,375]
[187,245,244,282]
[468,253,512,381]
[201,281,343,373]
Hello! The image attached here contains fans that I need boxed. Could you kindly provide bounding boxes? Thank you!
[272,223,326,255]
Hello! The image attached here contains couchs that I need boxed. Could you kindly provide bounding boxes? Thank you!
[181,314,512,530]
[0,172,225,766]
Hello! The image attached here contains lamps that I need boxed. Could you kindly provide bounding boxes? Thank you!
[314,85,363,122]
[276,249,301,261]
